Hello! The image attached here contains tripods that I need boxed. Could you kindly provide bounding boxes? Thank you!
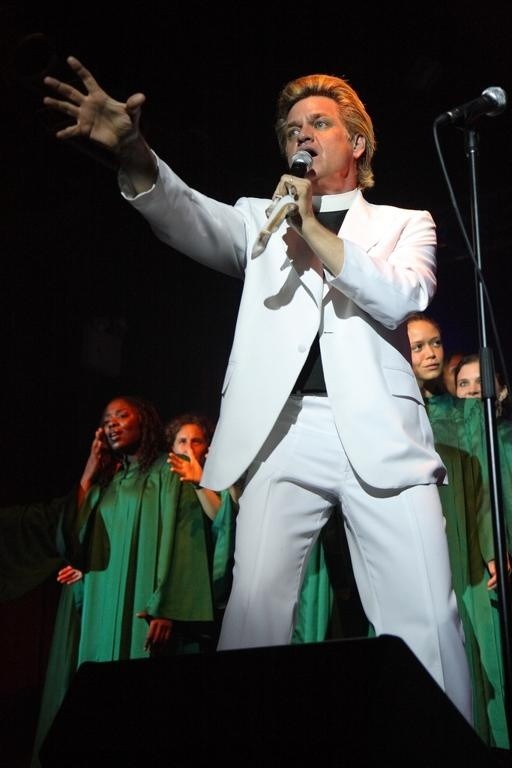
[462,129,511,763]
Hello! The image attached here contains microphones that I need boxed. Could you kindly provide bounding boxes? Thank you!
[436,84,508,128]
[289,149,313,197]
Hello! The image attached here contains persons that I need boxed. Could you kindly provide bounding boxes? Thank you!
[164,414,212,464]
[402,317,511,763]
[57,395,215,662]
[43,56,491,746]
[456,354,510,585]
[437,351,467,391]
